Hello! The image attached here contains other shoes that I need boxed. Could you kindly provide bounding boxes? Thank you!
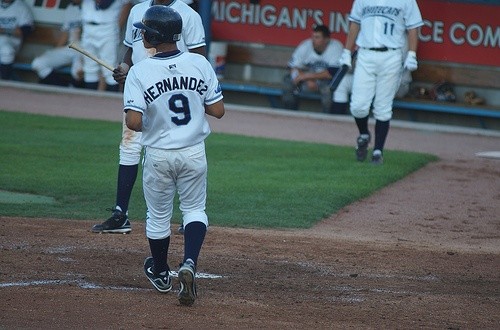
[356,134,370,161]
[373,149,382,162]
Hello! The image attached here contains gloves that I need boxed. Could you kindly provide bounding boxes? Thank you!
[338,48,351,69]
[404,51,417,71]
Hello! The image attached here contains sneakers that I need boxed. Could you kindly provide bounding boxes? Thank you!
[143,256,172,292]
[92,207,132,232]
[178,262,197,306]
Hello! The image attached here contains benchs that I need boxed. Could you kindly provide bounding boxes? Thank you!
[10,27,500,129]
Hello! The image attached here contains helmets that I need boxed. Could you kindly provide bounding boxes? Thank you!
[133,5,182,43]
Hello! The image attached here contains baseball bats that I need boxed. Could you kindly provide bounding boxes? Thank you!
[68,41,127,80]
[328,47,360,92]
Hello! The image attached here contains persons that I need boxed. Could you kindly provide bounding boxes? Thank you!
[31,0,84,89]
[71,0,131,91]
[124,4,226,307]
[338,0,424,165]
[91,0,209,234]
[0,0,36,80]
[280,25,344,113]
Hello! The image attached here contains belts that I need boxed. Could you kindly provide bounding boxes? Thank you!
[369,47,396,51]
